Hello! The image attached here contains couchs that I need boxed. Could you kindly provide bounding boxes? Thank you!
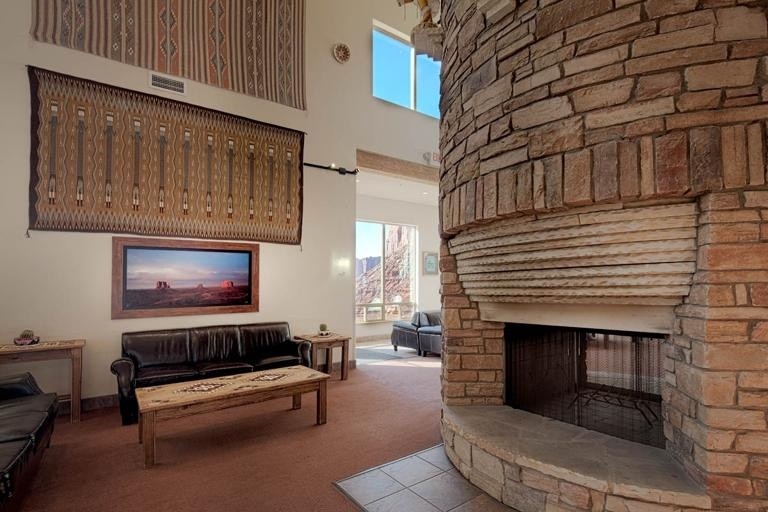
[2,371,60,511]
[109,321,312,427]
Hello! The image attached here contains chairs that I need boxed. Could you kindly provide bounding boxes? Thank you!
[389,310,444,357]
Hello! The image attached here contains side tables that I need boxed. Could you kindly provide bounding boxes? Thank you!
[294,332,352,380]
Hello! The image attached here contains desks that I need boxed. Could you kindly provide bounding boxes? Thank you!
[0,336,86,423]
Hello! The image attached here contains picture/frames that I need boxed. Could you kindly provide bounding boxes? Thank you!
[112,235,260,320]
